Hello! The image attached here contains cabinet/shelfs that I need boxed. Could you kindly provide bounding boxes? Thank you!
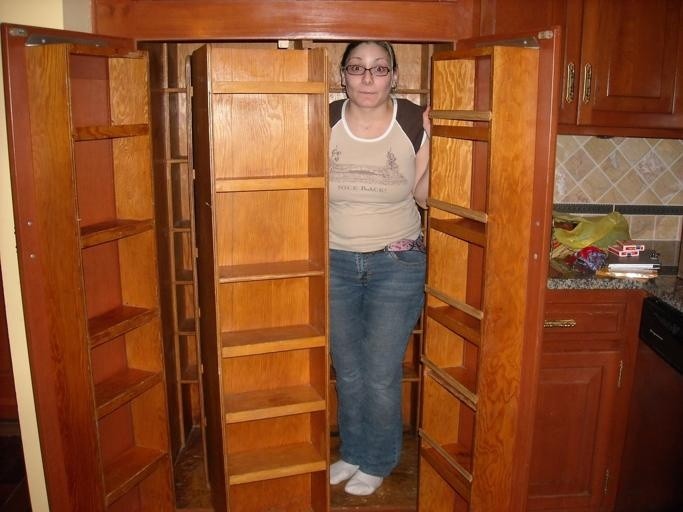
[620,272,683,510]
[0,20,562,512]
[86,0,481,39]
[532,269,650,511]
[482,3,682,139]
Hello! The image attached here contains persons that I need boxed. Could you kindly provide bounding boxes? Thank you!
[329,40,431,496]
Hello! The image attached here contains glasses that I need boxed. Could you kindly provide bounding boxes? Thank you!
[343,65,392,76]
